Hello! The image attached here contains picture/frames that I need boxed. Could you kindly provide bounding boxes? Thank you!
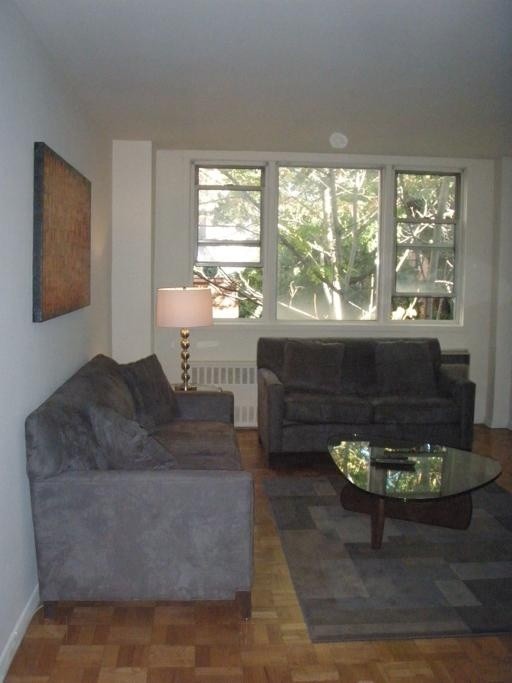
[34,138,93,324]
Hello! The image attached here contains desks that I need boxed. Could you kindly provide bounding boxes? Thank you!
[170,383,222,393]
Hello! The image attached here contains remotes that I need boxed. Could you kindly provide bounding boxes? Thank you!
[370,454,408,460]
[375,459,416,467]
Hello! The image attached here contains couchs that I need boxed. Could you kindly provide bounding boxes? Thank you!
[24,351,261,624]
[255,334,476,467]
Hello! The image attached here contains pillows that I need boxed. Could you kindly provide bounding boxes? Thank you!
[86,401,180,470]
[117,353,184,429]
[372,335,444,397]
[280,339,349,398]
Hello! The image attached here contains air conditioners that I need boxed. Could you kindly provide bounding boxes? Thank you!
[187,357,257,428]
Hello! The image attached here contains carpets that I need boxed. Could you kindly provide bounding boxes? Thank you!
[256,472,511,644]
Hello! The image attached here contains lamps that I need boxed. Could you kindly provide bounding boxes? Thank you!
[154,286,223,389]
